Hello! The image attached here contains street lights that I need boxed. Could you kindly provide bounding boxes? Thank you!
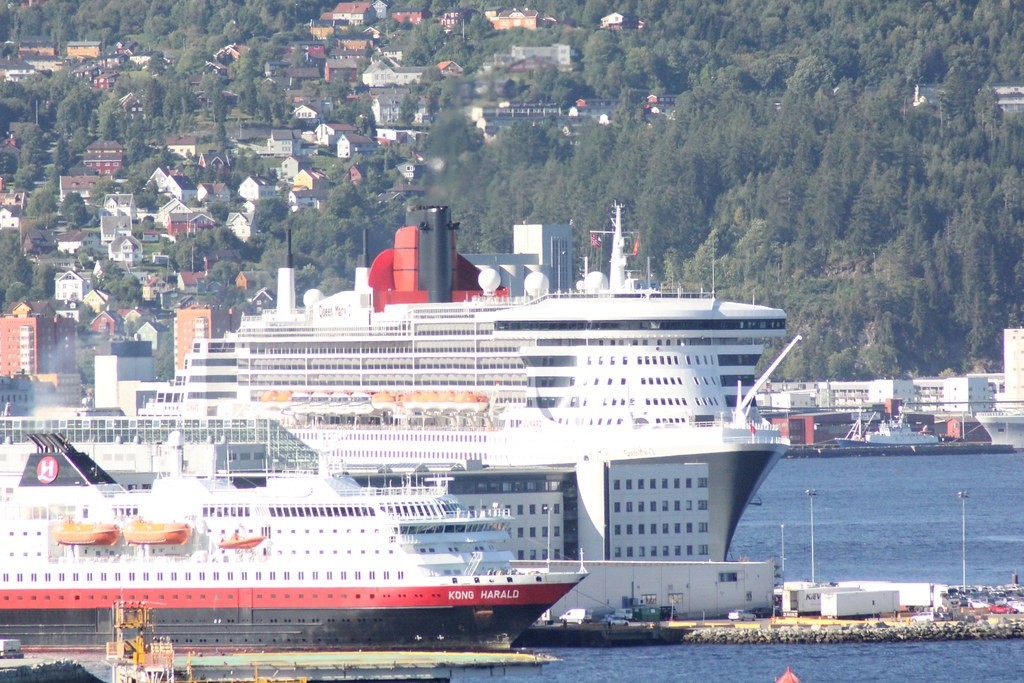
[780,523,786,588]
[958,490,970,594]
[804,490,818,583]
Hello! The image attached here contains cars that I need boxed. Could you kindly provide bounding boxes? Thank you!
[910,587,1024,623]
[727,608,756,622]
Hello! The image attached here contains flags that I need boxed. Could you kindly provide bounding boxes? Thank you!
[591,233,602,248]
[633,234,640,256]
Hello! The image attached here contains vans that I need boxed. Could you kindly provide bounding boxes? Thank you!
[558,607,634,627]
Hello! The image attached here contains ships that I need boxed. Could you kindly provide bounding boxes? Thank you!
[0,194,804,562]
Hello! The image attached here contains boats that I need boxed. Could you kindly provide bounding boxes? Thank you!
[835,403,940,448]
[0,428,592,654]
